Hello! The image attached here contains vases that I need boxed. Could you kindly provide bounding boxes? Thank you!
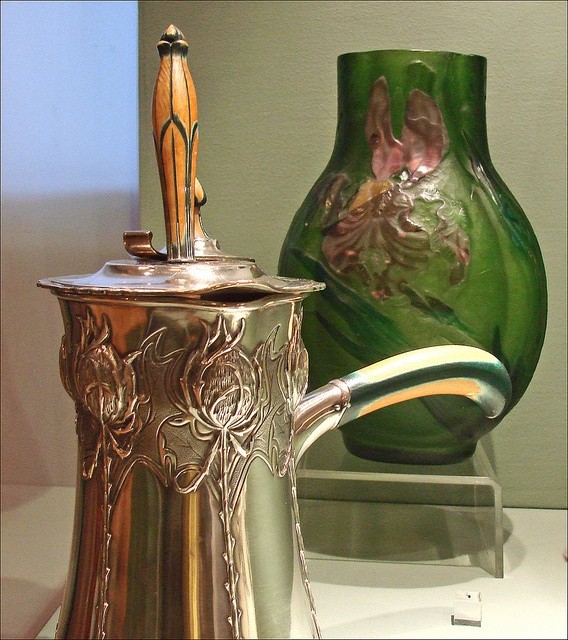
[274,48,551,467]
